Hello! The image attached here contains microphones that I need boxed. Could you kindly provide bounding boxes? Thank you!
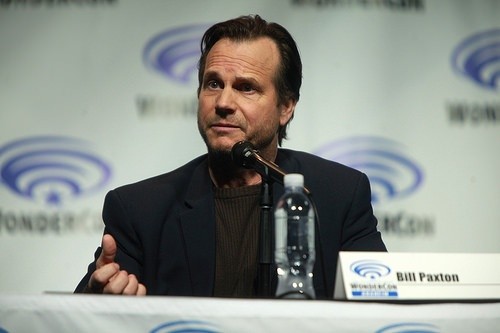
[231,140,313,200]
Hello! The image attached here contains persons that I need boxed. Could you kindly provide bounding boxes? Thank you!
[72,15,390,302]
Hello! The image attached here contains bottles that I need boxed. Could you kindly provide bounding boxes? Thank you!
[273,173,318,299]
